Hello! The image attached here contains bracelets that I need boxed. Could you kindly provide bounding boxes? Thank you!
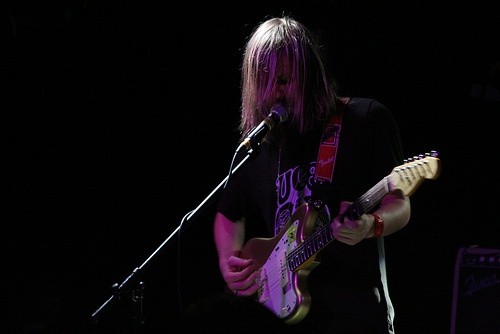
[369,213,385,239]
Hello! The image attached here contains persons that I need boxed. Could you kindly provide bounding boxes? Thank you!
[213,18,412,334]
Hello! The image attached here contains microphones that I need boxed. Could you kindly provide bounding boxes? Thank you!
[236,104,289,153]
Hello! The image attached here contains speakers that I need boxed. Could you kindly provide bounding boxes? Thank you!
[451,248,499,334]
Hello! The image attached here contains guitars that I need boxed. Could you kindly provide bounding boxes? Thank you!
[239,150,441,324]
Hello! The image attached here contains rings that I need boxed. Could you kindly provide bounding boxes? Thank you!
[235,289,240,295]
